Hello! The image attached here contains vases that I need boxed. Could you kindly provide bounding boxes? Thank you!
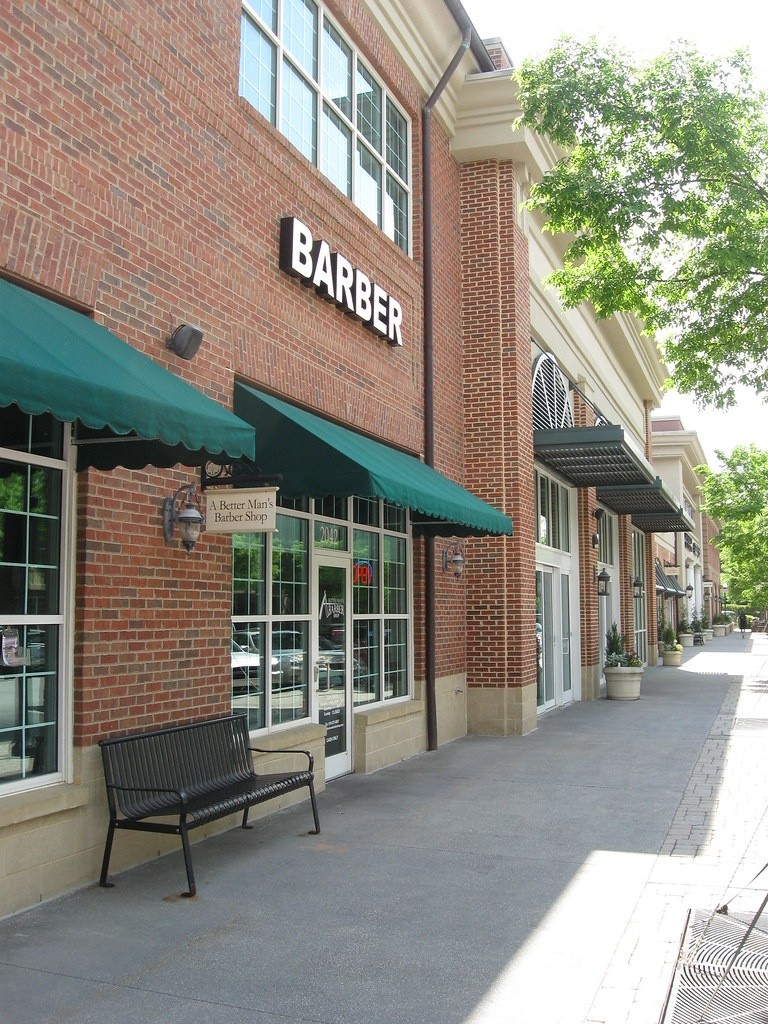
[603,665,644,700]
[663,650,682,666]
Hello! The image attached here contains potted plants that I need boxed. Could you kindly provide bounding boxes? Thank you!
[677,614,734,646]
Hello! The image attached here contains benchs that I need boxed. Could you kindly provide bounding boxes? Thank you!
[98,712,321,898]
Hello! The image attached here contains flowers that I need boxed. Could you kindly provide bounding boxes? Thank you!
[662,624,683,652]
[603,622,642,666]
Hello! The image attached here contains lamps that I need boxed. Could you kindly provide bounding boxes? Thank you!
[162,480,204,554]
[633,577,643,598]
[593,507,604,519]
[704,590,722,602]
[442,540,463,581]
[685,583,693,598]
[596,568,611,596]
[165,324,203,361]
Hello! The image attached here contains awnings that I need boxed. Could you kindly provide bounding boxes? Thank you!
[533,338,694,532]
[0,279,254,470]
[655,565,686,599]
[233,376,510,538]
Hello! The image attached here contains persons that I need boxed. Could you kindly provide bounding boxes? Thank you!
[740,610,746,632]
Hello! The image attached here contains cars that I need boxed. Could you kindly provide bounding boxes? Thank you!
[232,637,284,692]
[233,627,362,686]
